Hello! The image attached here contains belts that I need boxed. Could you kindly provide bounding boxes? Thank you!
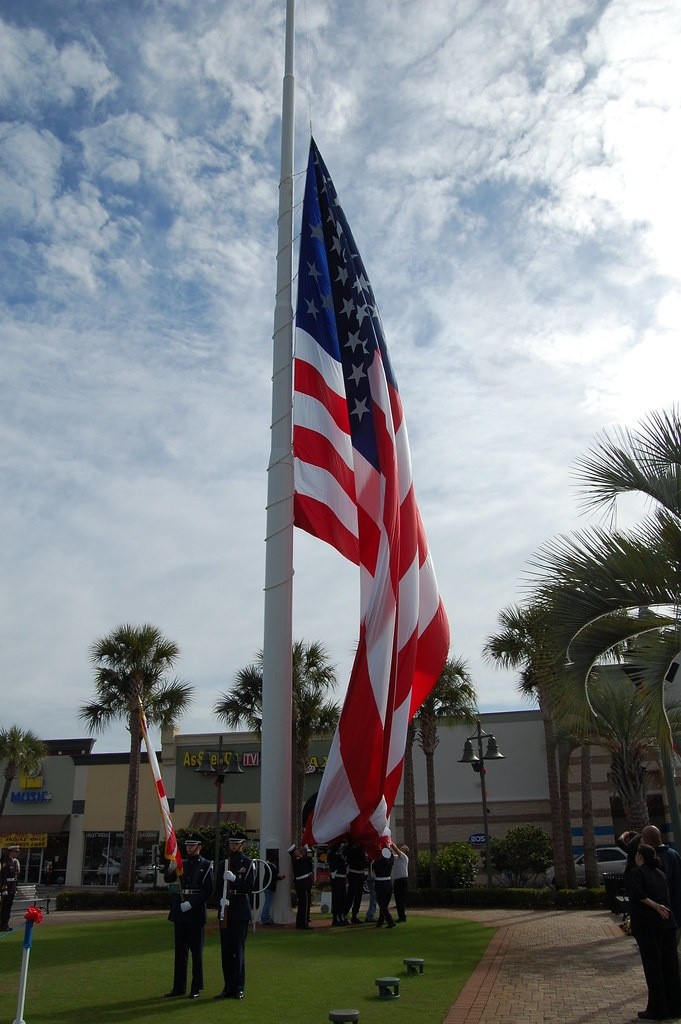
[6,878,18,881]
[374,876,391,880]
[330,872,347,877]
[230,889,246,894]
[296,872,312,880]
[177,889,201,895]
[348,867,367,874]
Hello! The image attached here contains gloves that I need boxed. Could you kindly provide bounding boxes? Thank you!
[304,844,309,850]
[181,901,191,912]
[15,891,17,895]
[2,891,9,896]
[220,898,229,906]
[169,861,177,871]
[223,871,236,882]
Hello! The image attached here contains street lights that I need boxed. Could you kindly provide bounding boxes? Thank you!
[457,721,507,890]
[193,736,245,891]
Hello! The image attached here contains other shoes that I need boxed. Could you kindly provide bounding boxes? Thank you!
[385,924,396,928]
[297,924,314,930]
[395,917,406,923]
[333,919,377,926]
[376,921,384,927]
[1,928,13,931]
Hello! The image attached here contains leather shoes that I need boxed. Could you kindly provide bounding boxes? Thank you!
[215,992,230,998]
[164,991,186,998]
[189,991,199,998]
[235,990,244,999]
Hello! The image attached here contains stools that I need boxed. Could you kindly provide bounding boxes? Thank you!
[403,958,424,975]
[374,976,401,999]
[328,1009,360,1024]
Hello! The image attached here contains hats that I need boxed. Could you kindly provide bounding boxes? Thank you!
[184,832,206,844]
[8,845,19,849]
[382,848,391,858]
[287,844,295,852]
[228,830,247,844]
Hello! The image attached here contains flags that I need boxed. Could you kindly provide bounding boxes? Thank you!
[139,707,185,880]
[288,138,452,859]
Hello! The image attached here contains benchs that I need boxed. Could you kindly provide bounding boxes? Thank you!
[0,885,40,907]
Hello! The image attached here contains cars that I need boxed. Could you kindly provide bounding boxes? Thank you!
[546,847,628,888]
[138,862,157,882]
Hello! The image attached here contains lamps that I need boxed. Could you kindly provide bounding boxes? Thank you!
[73,814,79,817]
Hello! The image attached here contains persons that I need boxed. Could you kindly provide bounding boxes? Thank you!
[164,832,214,999]
[287,843,313,929]
[261,852,287,927]
[214,831,254,1000]
[45,859,53,887]
[0,845,20,932]
[328,840,409,929]
[618,826,681,1020]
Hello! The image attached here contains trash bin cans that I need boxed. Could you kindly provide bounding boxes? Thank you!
[601,872,624,913]
